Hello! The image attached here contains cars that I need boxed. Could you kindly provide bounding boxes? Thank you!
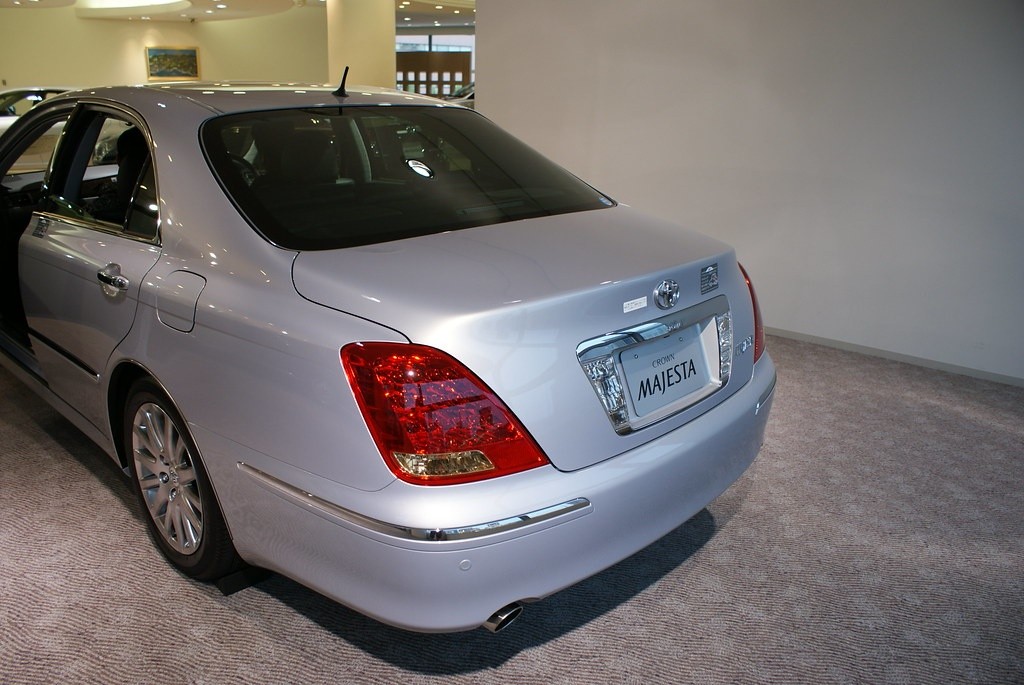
[1,88,135,174]
[1,80,776,635]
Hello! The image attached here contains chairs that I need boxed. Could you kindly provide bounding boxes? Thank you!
[114,148,154,231]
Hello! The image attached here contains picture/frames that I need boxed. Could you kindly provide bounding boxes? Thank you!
[144,45,202,83]
